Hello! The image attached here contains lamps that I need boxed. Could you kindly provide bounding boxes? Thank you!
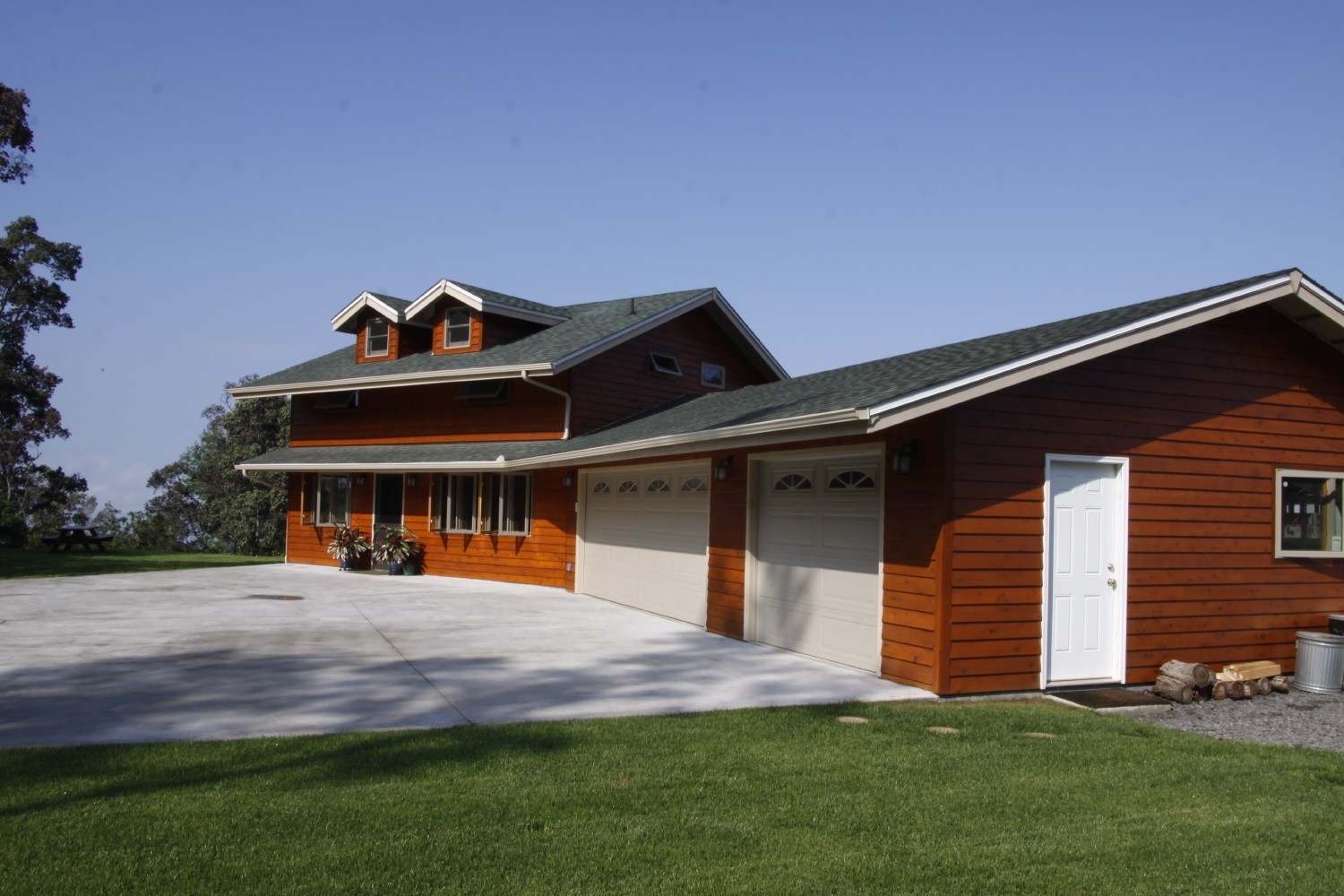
[356,474,367,487]
[563,470,578,486]
[892,439,918,476]
[714,455,733,482]
[408,473,419,486]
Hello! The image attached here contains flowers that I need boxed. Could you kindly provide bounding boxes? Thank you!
[322,522,372,560]
[372,523,421,564]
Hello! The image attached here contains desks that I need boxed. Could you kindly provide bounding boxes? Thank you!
[54,525,106,554]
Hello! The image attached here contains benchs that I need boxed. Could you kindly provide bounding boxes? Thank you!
[41,533,113,545]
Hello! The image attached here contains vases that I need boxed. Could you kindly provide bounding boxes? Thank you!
[401,551,421,575]
[339,555,357,572]
[388,559,403,575]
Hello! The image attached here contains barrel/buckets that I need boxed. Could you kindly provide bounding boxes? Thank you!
[1294,630,1344,696]
